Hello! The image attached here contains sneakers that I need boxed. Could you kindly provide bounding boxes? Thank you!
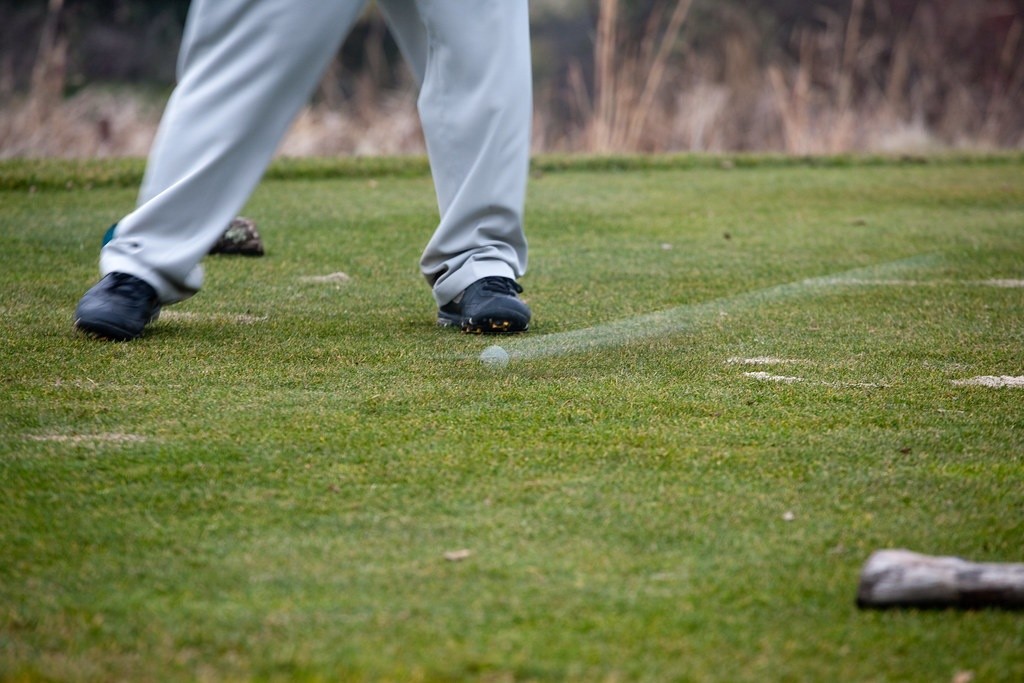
[73,272,162,337]
[439,277,531,335]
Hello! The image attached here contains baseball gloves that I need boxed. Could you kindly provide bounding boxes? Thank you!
[207,217,264,254]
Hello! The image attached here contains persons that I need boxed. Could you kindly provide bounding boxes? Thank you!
[75,1,536,340]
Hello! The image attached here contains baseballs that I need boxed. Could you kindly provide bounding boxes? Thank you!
[481,345,509,372]
